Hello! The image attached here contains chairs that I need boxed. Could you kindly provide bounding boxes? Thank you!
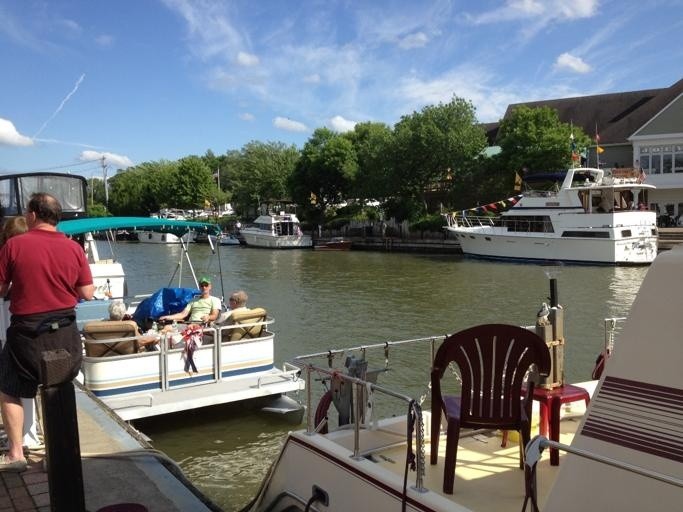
[156,291,225,325]
[203,307,267,343]
[430,323,551,503]
[88,322,159,358]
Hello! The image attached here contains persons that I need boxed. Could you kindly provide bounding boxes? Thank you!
[158,276,220,324]
[108,302,142,335]
[626,198,648,212]
[217,290,252,323]
[0,193,93,470]
[0,215,28,242]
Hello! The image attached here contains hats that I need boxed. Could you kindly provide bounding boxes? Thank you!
[199,276,210,285]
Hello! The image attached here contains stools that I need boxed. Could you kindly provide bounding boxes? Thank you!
[502,370,590,467]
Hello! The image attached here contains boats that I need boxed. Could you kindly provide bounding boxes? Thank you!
[447,167,659,264]
[239,199,313,247]
[241,247,682,512]
[0,170,127,303]
[135,222,241,246]
[316,236,350,249]
[56,216,307,422]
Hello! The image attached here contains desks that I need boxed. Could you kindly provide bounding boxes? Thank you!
[143,325,189,350]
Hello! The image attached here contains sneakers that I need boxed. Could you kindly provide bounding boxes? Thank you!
[0,454,27,472]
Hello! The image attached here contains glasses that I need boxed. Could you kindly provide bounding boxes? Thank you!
[200,284,208,287]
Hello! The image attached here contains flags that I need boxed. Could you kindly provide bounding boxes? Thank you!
[513,171,522,192]
[639,168,646,182]
